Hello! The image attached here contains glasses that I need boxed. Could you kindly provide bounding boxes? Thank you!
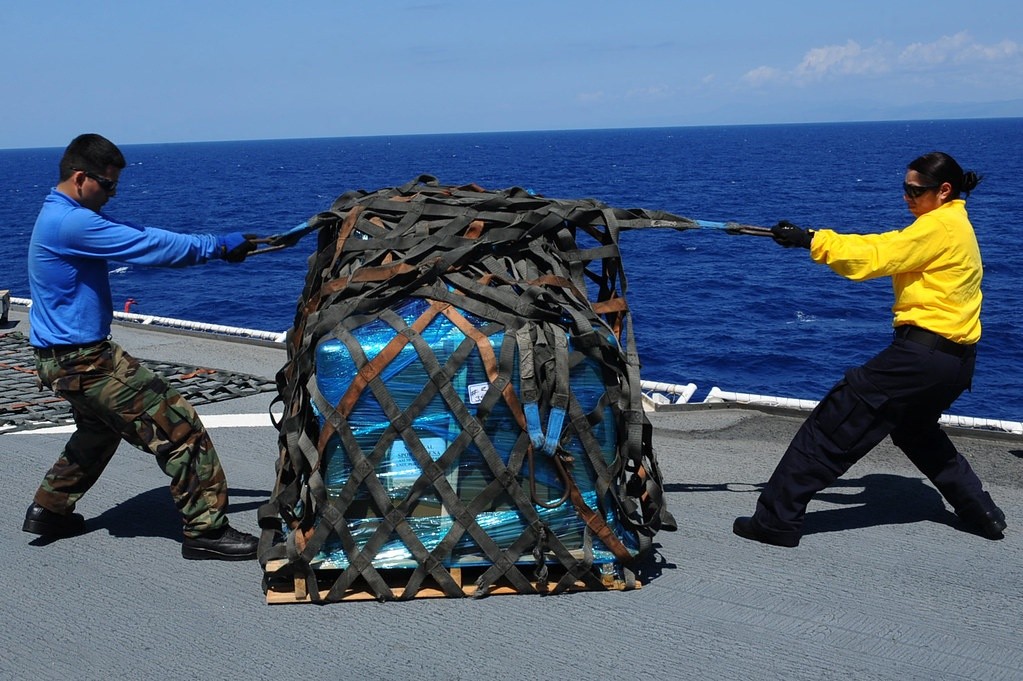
[903,180,938,198]
[71,167,118,193]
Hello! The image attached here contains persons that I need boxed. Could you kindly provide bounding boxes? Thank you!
[733,152,1007,547]
[22,134,259,562]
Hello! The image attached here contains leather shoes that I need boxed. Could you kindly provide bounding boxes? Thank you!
[181,524,259,561]
[23,501,84,535]
[956,492,1007,536]
[734,517,801,547]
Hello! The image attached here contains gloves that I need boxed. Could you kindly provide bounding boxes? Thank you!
[213,232,258,265]
[770,220,812,250]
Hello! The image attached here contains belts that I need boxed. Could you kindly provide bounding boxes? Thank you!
[34,338,107,358]
[895,325,978,358]
